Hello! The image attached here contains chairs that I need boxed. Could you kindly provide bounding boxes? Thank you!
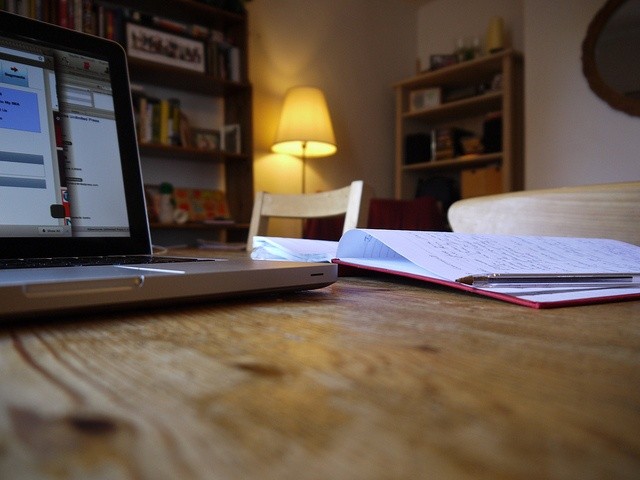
[247,181,368,258]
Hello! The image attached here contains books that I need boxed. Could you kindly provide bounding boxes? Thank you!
[134,96,183,146]
[1,1,117,43]
[205,36,230,81]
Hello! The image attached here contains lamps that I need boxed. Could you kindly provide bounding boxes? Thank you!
[270,85,337,195]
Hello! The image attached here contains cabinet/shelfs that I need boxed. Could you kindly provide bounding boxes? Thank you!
[2,1,254,248]
[386,48,525,230]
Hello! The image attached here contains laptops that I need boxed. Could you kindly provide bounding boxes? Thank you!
[0,12,339,324]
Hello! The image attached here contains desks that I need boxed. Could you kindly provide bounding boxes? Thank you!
[0,250,638,480]
